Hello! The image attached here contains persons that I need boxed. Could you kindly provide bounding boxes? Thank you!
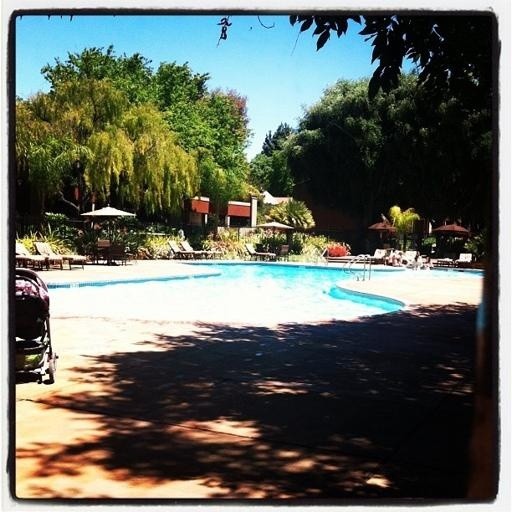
[174,227,186,241]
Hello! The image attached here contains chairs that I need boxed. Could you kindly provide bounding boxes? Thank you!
[243,242,277,263]
[366,243,475,269]
[167,241,224,262]
[15,241,136,272]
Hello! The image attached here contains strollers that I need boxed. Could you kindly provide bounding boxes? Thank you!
[15,268,60,384]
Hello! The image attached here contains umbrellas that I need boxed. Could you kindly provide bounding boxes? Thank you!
[431,224,468,258]
[367,220,395,246]
[253,221,295,254]
[80,205,138,262]
